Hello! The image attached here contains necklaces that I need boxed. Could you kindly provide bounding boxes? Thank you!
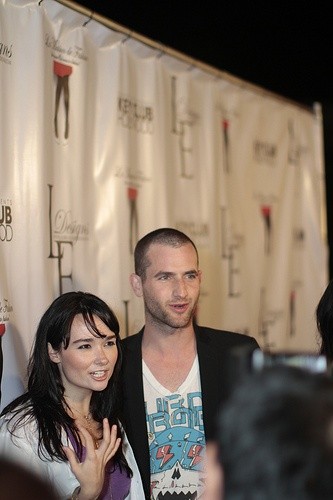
[69,405,90,421]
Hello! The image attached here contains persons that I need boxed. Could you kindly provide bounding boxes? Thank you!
[199,365,333,500]
[0,292,145,500]
[117,228,263,500]
